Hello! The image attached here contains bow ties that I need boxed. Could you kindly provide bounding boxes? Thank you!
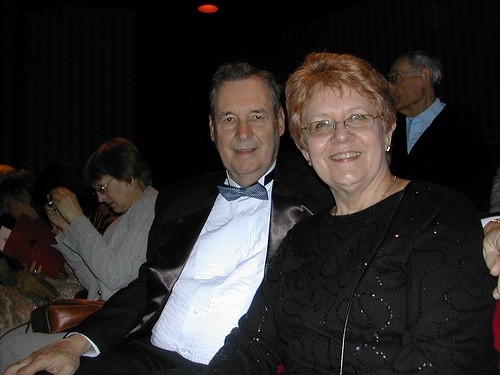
[216,181,269,202]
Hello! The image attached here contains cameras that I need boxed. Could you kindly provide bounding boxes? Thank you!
[46,194,54,206]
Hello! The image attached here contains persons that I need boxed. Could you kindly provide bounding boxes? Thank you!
[3,62,500,375]
[206,52,500,375]
[388,54,496,208]
[0,136,159,375]
[0,139,165,331]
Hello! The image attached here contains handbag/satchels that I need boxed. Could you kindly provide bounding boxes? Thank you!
[25,299,106,334]
[12,271,60,303]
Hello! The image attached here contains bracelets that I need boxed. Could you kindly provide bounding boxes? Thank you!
[492,218,500,223]
[381,176,396,199]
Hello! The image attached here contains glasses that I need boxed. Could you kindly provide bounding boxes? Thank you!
[300,113,384,139]
[92,177,114,199]
[385,71,420,85]
